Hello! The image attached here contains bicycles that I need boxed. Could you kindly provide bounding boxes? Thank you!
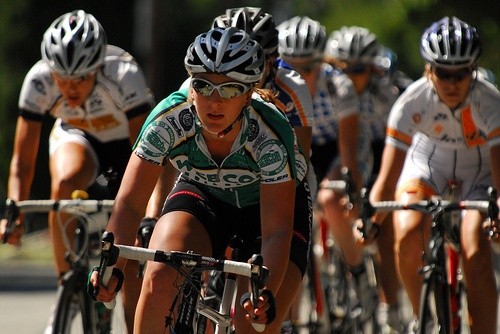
[3,165,499,334]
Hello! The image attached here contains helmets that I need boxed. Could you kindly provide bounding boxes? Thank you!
[212,6,279,55]
[369,46,397,85]
[276,16,327,63]
[419,15,481,68]
[324,26,379,64]
[184,28,266,83]
[40,8,106,76]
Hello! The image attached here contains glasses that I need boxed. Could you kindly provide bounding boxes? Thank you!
[328,64,372,75]
[290,60,319,74]
[190,77,251,99]
[429,62,473,83]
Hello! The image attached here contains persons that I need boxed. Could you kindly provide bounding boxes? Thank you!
[135,6,313,260]
[0,9,154,334]
[276,17,416,334]
[353,16,500,334]
[88,27,313,334]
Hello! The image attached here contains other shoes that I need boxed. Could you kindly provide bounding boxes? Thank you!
[377,303,405,334]
[407,318,436,334]
